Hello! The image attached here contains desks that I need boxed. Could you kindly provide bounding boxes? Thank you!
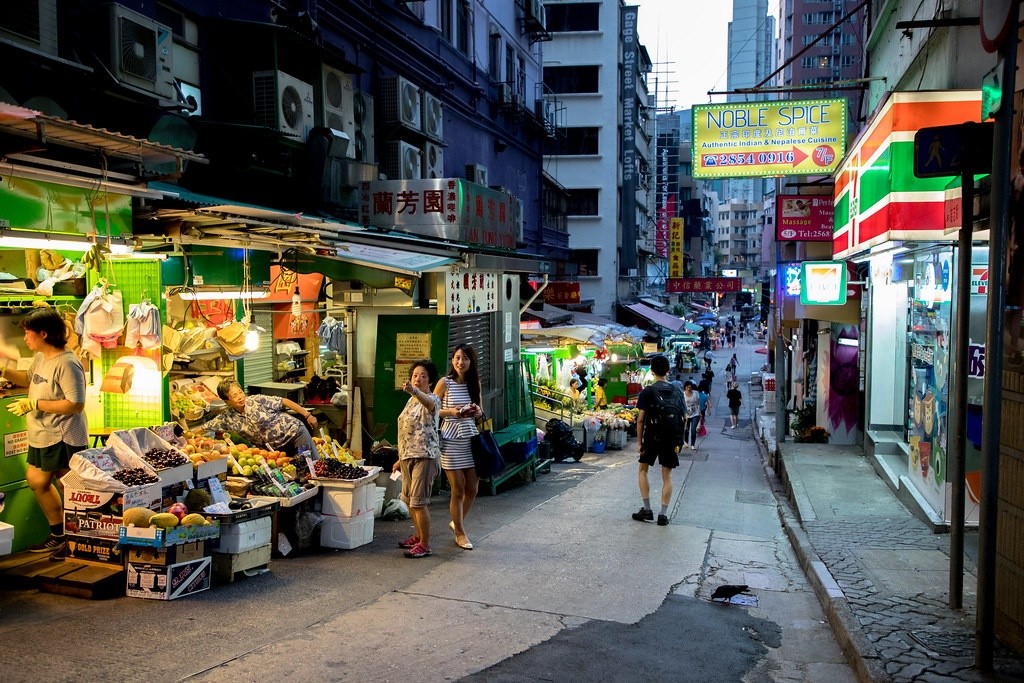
[248,381,316,437]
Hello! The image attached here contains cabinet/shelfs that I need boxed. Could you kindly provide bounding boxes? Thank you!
[474,423,536,495]
[166,285,237,432]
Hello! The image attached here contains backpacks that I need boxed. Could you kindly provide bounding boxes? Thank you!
[646,386,683,447]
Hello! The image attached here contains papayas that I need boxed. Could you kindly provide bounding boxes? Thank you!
[123,508,204,527]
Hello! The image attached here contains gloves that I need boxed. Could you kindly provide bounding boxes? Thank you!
[7,398,38,417]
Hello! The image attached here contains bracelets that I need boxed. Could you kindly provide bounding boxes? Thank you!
[305,412,311,419]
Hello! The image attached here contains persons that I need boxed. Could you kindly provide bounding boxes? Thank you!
[726,382,743,428]
[632,355,687,526]
[720,312,745,349]
[192,378,320,461]
[433,345,483,549]
[683,381,700,449]
[725,364,732,389]
[593,377,608,410]
[0,307,88,561]
[673,347,716,435]
[566,379,580,400]
[730,353,739,375]
[393,359,441,558]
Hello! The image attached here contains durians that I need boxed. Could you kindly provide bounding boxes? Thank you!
[184,486,210,512]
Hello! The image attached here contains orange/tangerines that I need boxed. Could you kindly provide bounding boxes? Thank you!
[170,395,196,414]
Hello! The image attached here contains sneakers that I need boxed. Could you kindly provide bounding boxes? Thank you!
[50,544,65,561]
[28,531,65,552]
[400,535,421,548]
[404,540,432,557]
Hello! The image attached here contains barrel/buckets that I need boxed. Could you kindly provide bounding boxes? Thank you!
[592,440,605,454]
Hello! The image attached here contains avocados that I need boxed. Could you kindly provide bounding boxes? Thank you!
[286,477,315,491]
[229,501,252,511]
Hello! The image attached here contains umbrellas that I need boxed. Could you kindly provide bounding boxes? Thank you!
[682,322,704,334]
[694,320,716,327]
[696,310,717,320]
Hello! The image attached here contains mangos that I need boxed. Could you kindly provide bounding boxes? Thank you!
[177,432,288,477]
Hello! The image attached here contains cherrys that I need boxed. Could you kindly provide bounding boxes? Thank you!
[112,465,158,486]
[305,458,368,480]
[142,447,186,468]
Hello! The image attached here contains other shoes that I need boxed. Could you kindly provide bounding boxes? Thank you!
[691,445,695,450]
[449,521,455,532]
[737,424,738,426]
[684,440,688,448]
[632,507,653,520]
[455,535,473,549]
[657,515,669,525]
[731,426,734,428]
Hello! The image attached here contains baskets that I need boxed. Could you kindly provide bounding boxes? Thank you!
[60,469,85,490]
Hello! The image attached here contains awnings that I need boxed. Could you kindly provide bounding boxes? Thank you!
[0,102,209,181]
[140,209,328,256]
[624,302,684,334]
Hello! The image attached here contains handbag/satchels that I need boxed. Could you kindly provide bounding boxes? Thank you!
[699,418,707,436]
[471,412,505,479]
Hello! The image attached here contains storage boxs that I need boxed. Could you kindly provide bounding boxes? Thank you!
[39,277,86,295]
[751,372,776,412]
[61,421,387,598]
[613,342,664,408]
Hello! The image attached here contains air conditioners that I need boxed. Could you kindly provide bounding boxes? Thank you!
[636,224,642,238]
[498,84,555,137]
[628,269,637,277]
[82,2,174,107]
[254,64,444,179]
[636,172,643,190]
[466,163,523,244]
[525,0,546,41]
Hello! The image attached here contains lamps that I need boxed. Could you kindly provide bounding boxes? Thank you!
[412,278,429,308]
[817,328,835,334]
[291,272,303,316]
[764,326,768,329]
[593,345,609,371]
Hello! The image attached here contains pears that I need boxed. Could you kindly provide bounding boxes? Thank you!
[311,435,356,464]
